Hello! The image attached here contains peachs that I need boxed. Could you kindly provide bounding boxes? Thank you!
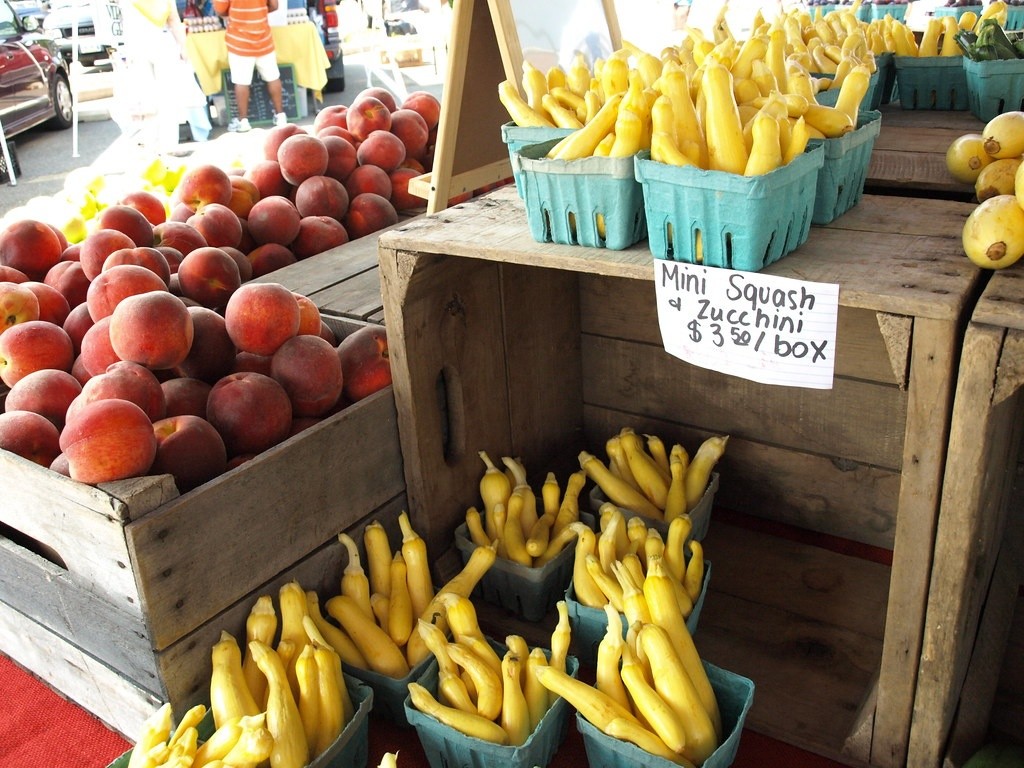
[0,85,512,496]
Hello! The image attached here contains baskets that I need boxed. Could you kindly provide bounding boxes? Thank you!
[501,3,1023,274]
[104,470,754,768]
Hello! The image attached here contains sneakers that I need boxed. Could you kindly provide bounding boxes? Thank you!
[227,118,251,132]
[272,112,287,125]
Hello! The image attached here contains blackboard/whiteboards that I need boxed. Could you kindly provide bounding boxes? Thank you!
[220,60,301,127]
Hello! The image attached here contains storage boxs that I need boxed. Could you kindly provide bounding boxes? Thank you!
[381,102,1024,767]
[501,4,1024,272]
[0,314,410,745]
[104,475,755,768]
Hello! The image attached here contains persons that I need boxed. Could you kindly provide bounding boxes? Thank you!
[213,0,287,131]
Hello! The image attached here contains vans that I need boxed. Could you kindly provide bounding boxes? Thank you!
[180,0,346,92]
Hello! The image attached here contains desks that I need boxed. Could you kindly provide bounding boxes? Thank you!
[187,21,332,127]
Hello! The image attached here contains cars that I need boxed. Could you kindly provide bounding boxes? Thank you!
[357,0,441,35]
[15,1,106,66]
[0,0,74,183]
